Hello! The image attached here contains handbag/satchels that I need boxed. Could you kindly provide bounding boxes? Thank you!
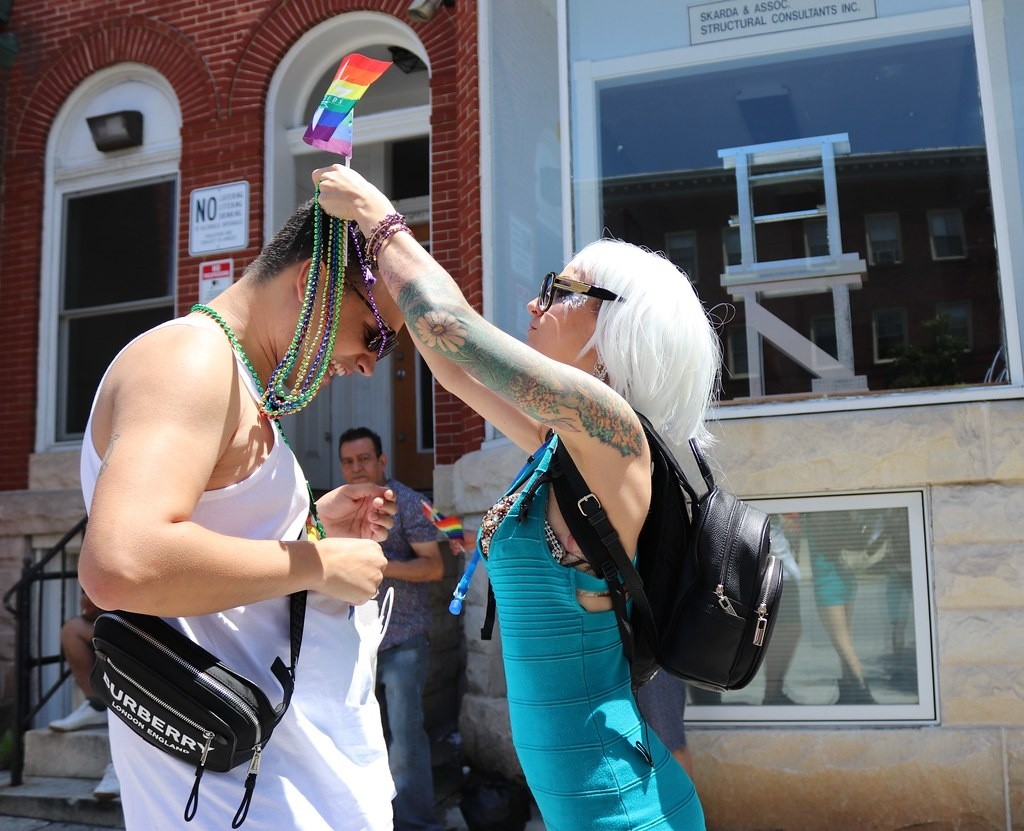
[87,606,280,774]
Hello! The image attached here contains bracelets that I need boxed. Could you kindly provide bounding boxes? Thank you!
[362,212,413,268]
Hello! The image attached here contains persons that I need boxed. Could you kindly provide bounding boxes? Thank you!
[314,162,716,828]
[762,512,801,705]
[337,426,442,831]
[805,512,877,705]
[78,194,395,831]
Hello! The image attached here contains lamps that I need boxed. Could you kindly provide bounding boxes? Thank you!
[87,109,145,155]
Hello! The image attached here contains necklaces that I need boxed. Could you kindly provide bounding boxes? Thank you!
[260,176,386,420]
[188,305,327,540]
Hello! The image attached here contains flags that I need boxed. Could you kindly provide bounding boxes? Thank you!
[302,53,392,158]
[422,504,466,540]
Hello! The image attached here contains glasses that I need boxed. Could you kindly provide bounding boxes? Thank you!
[539,272,626,312]
[346,277,399,362]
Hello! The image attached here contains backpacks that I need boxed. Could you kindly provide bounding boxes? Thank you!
[547,411,785,699]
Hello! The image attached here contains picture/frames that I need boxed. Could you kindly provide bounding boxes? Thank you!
[802,217,829,257]
[926,207,969,262]
[721,226,742,272]
[865,211,905,267]
[726,322,767,379]
[664,230,699,285]
[871,306,910,364]
[811,315,849,373]
[934,299,974,354]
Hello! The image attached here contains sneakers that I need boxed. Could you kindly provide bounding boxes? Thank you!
[49,699,107,730]
[93,764,121,799]
